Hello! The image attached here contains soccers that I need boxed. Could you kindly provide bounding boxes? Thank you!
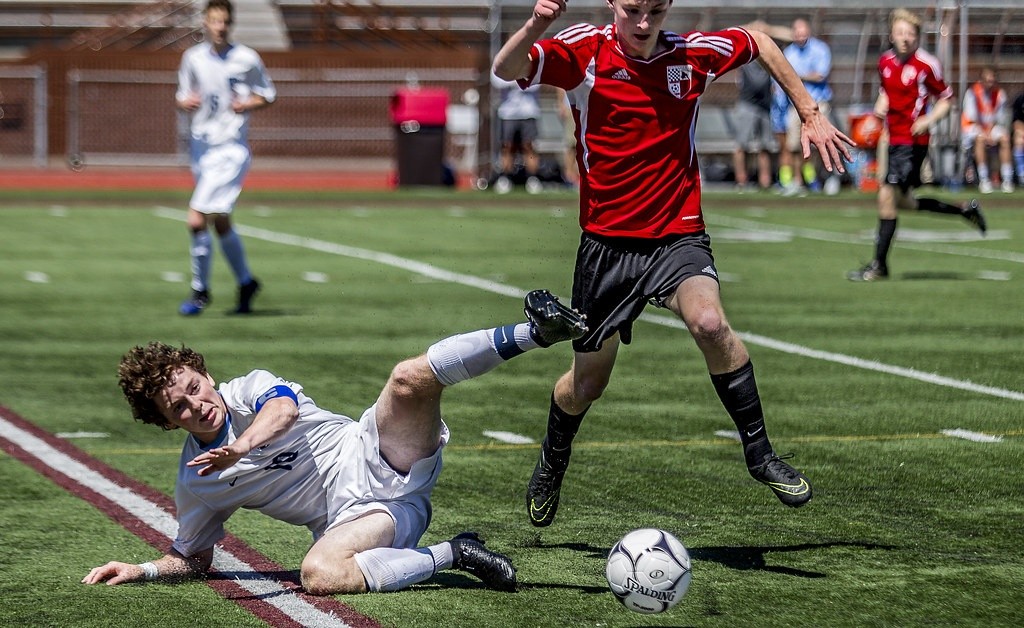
[605,526,692,612]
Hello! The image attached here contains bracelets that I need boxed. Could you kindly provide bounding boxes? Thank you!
[136,562,159,582]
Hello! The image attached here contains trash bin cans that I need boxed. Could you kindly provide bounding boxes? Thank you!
[388,89,451,190]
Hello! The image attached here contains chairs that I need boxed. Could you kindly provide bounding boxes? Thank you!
[535,97,964,188]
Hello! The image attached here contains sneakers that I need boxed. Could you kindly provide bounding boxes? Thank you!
[747,452,813,507]
[846,264,885,281]
[523,288,588,343]
[963,201,986,231]
[525,437,571,526]
[453,530,517,589]
[236,281,256,312]
[178,292,211,314]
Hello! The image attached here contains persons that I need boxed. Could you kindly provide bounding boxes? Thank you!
[488,16,1023,200]
[492,1,856,527]
[841,6,987,283]
[173,1,278,318]
[81,288,590,599]
